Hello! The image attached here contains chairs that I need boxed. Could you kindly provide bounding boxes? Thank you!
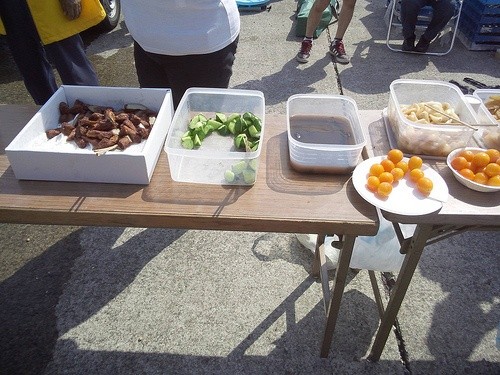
[387,0,464,56]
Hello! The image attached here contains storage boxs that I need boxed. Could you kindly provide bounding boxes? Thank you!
[5,78,500,185]
[386,0,500,50]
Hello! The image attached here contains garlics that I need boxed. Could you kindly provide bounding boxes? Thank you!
[391,101,466,158]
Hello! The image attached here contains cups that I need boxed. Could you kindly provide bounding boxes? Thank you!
[461,93,482,115]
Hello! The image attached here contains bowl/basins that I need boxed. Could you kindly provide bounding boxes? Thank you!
[446,146,500,193]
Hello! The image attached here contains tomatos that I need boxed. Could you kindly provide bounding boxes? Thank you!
[451,149,500,187]
[367,149,434,196]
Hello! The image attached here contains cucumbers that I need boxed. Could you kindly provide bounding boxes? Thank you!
[179,112,262,185]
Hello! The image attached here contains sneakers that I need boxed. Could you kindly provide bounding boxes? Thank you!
[415,35,430,52]
[403,35,415,50]
[330,40,350,62]
[297,39,313,63]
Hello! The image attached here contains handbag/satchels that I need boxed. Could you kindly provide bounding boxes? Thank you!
[296,0,340,38]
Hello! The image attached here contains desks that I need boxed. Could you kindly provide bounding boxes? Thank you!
[0,105,380,358]
[356,108,500,362]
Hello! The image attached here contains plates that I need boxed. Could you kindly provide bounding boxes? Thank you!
[352,154,449,216]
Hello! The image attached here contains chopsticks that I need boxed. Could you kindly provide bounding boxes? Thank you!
[424,104,498,131]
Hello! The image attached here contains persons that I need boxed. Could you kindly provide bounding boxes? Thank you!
[0,0,106,106]
[400,0,457,53]
[122,0,240,111]
[297,0,357,64]
